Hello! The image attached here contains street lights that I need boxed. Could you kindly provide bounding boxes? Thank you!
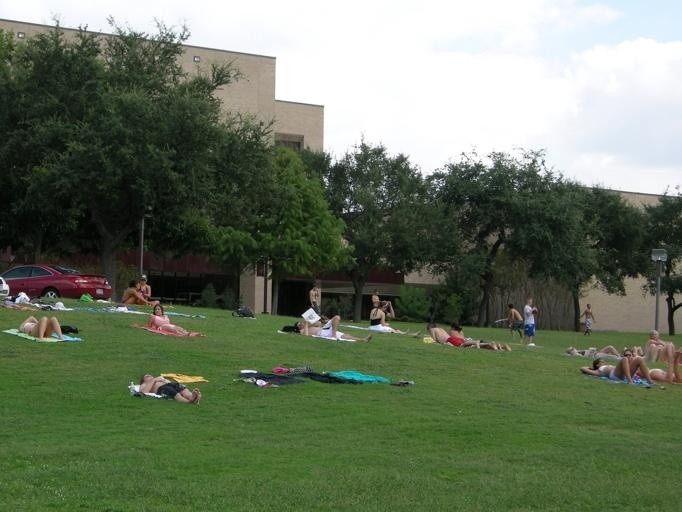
[139,206,153,275]
[649,247,668,332]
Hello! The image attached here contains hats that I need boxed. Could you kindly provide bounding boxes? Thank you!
[141,275,147,280]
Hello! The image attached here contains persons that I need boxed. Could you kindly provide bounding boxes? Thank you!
[579,303,598,336]
[380,300,398,322]
[372,288,383,309]
[281,315,372,343]
[136,273,152,304]
[310,282,321,314]
[427,322,499,351]
[449,319,512,352]
[567,328,682,387]
[148,304,206,339]
[119,279,160,308]
[370,301,421,338]
[19,316,67,341]
[523,295,537,347]
[139,371,202,405]
[506,302,523,338]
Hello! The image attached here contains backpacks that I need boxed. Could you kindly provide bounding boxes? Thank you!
[232,306,254,317]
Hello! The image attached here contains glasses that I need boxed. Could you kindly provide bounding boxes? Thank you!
[624,354,631,356]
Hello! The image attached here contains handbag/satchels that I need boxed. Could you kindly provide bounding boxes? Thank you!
[60,325,78,334]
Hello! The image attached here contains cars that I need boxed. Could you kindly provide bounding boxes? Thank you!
[0,261,113,301]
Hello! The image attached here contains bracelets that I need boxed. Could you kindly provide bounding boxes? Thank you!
[311,304,313,306]
